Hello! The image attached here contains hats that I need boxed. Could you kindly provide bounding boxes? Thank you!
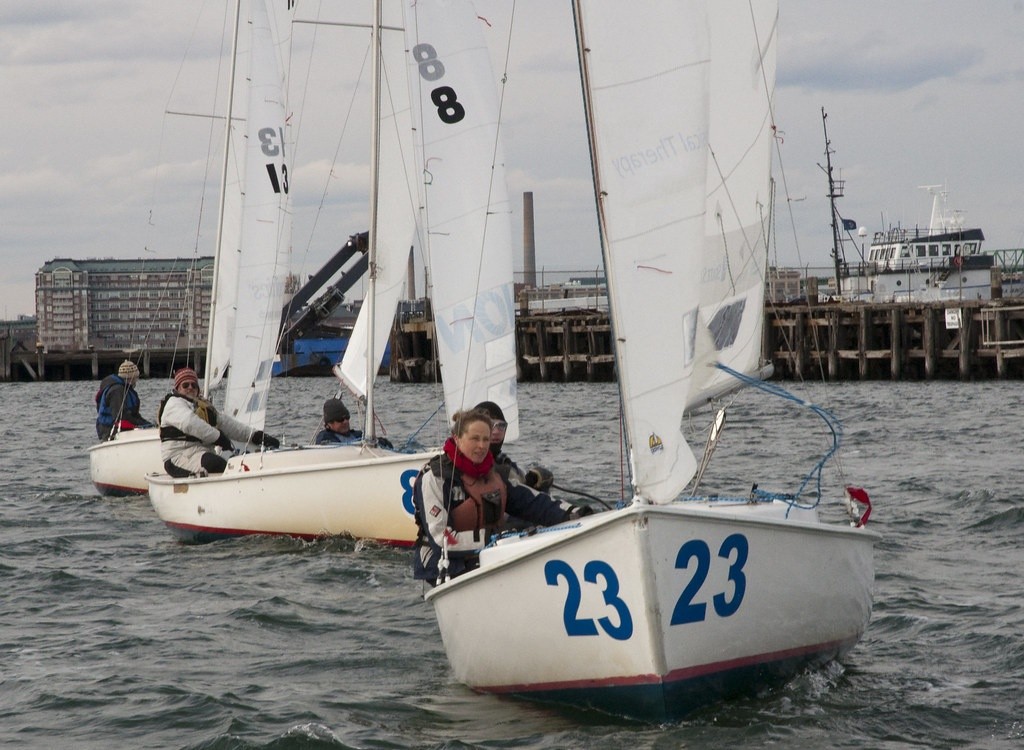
[324,398,349,422]
[474,401,505,421]
[174,368,198,390]
[118,360,139,378]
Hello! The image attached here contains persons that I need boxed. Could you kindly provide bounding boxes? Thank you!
[158,368,279,478]
[315,399,394,451]
[95,359,154,444]
[413,401,594,588]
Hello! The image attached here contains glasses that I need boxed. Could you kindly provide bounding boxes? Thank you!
[491,420,507,431]
[336,415,350,422]
[182,382,198,388]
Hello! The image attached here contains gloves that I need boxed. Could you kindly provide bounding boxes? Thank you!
[564,505,593,521]
[252,431,279,449]
[491,528,517,539]
[529,466,554,494]
[212,429,235,451]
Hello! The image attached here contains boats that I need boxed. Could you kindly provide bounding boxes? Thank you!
[816,106,1024,303]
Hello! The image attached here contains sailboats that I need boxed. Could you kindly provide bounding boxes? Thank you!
[426,1,879,725]
[86,0,296,497]
[142,0,446,550]
